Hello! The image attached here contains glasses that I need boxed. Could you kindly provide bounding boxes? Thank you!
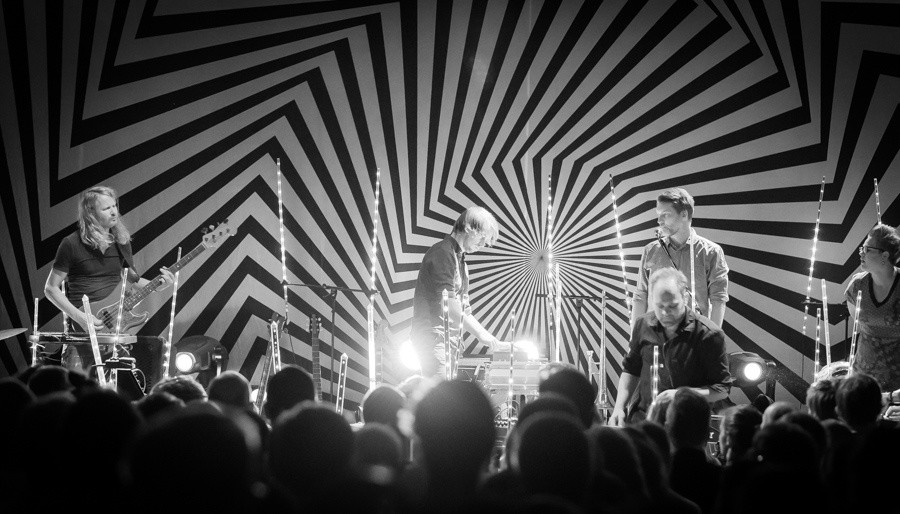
[858,246,884,254]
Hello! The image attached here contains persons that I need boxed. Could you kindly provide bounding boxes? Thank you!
[845,224,900,392]
[44,187,173,385]
[0,364,900,514]
[631,187,728,338]
[411,207,514,384]
[609,268,732,427]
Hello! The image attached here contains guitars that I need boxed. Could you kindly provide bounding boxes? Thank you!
[255,313,285,416]
[306,312,322,408]
[71,218,237,356]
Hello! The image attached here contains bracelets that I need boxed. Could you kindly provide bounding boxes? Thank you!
[890,391,894,404]
